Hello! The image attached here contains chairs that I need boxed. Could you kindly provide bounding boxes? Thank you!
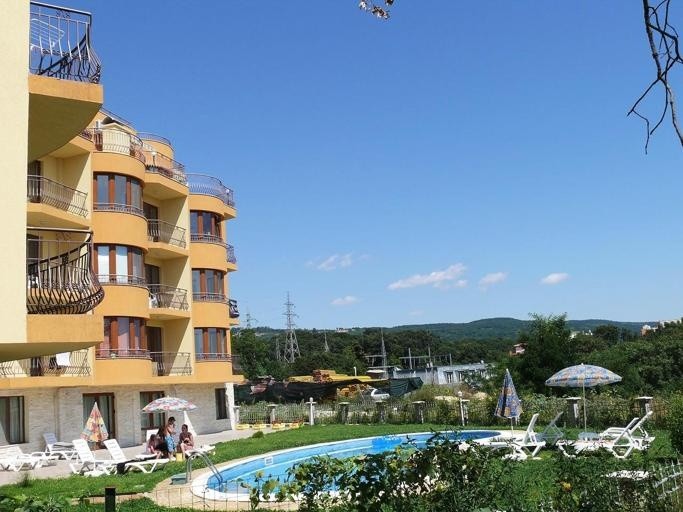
[60,439,118,478]
[512,412,565,446]
[42,432,80,464]
[554,417,639,460]
[480,413,546,462]
[103,439,169,473]
[147,428,215,460]
[0,443,60,472]
[578,403,656,451]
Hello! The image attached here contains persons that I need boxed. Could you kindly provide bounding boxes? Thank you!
[146,417,193,463]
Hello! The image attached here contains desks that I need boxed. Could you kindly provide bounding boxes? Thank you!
[600,470,650,509]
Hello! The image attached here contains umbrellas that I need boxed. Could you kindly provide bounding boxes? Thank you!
[544,362,622,434]
[80,401,109,472]
[495,367,524,436]
[144,395,196,418]
